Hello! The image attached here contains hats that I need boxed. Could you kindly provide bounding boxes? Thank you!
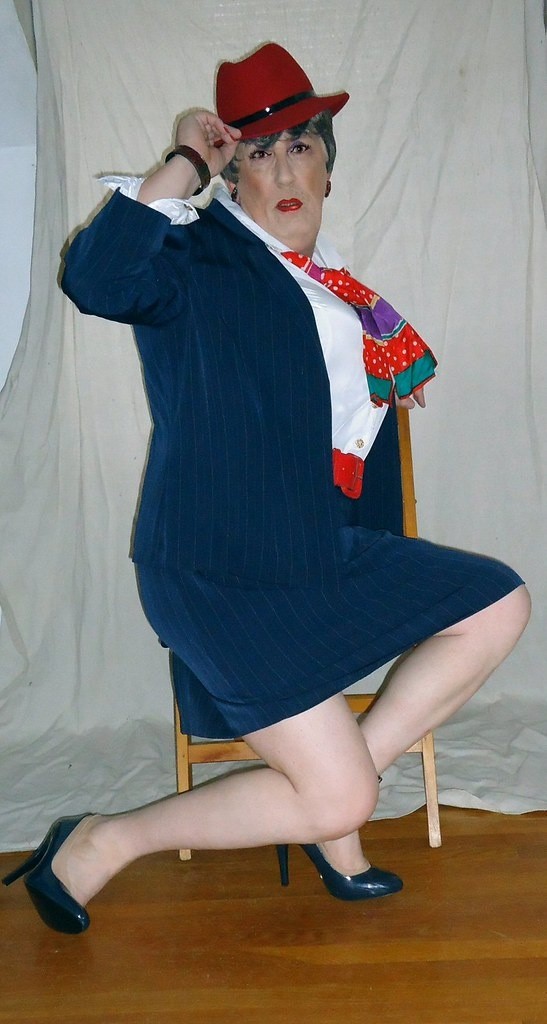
[212,43,351,146]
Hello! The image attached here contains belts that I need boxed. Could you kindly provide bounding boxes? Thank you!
[332,448,363,499]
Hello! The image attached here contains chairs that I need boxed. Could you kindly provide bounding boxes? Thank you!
[173,394,441,860]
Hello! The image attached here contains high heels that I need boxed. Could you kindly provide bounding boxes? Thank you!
[277,841,404,901]
[1,813,91,934]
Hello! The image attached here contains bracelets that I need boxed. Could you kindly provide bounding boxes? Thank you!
[165,145,211,196]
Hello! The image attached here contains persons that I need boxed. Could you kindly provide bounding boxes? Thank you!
[1,42,531,933]
